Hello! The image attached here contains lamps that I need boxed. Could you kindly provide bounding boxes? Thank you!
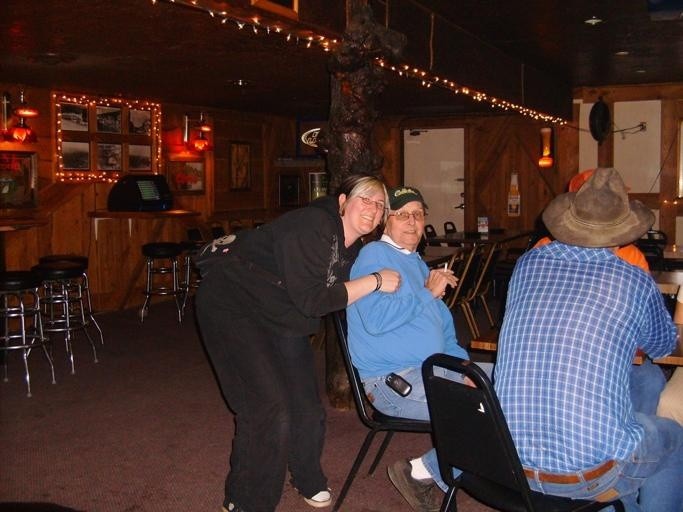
[537,124,558,170]
[1,86,39,134]
[182,111,212,150]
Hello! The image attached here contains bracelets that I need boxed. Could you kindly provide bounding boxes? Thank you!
[371,271,382,292]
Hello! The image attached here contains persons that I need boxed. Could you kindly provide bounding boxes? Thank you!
[345,186,494,512]
[656,283,683,426]
[193,170,401,512]
[494,166,683,511]
[534,171,668,511]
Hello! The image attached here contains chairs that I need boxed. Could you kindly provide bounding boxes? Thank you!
[333,310,431,510]
[417,221,531,341]
[421,354,626,511]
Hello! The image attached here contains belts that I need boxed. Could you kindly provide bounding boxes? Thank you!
[522,459,617,484]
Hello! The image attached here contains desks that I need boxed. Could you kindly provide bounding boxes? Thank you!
[472,228,682,365]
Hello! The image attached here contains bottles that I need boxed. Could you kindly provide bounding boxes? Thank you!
[506,171,522,218]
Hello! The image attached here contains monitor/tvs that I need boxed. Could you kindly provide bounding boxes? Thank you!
[136,180,160,201]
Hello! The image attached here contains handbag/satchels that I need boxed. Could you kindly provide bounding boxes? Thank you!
[195,224,256,274]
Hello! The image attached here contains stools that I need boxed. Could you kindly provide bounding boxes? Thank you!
[140,234,214,329]
[1,255,104,399]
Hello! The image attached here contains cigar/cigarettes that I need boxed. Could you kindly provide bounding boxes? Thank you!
[443,261,448,272]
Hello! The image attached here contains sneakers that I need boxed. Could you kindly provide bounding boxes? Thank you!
[222,497,242,511]
[288,476,332,507]
[387,457,442,511]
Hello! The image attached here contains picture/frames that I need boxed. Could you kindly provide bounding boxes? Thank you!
[277,172,300,208]
[228,140,256,192]
[0,149,39,210]
[48,88,164,185]
[159,155,206,200]
[306,171,330,204]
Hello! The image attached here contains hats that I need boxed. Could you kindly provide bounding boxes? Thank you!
[543,165,654,248]
[385,186,430,211]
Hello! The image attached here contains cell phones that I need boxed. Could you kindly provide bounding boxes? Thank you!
[385,372,412,397]
